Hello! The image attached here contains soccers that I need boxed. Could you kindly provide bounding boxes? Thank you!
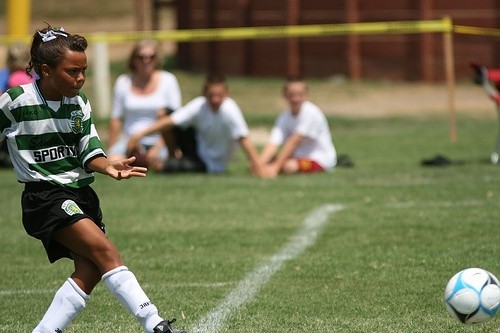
[442,267,500,327]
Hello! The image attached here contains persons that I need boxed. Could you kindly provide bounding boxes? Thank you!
[0,22,176,333]
[0,36,500,179]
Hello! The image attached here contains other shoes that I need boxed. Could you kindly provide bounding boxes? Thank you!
[153,318,186,333]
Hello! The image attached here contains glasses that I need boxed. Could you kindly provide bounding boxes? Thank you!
[137,54,155,61]
